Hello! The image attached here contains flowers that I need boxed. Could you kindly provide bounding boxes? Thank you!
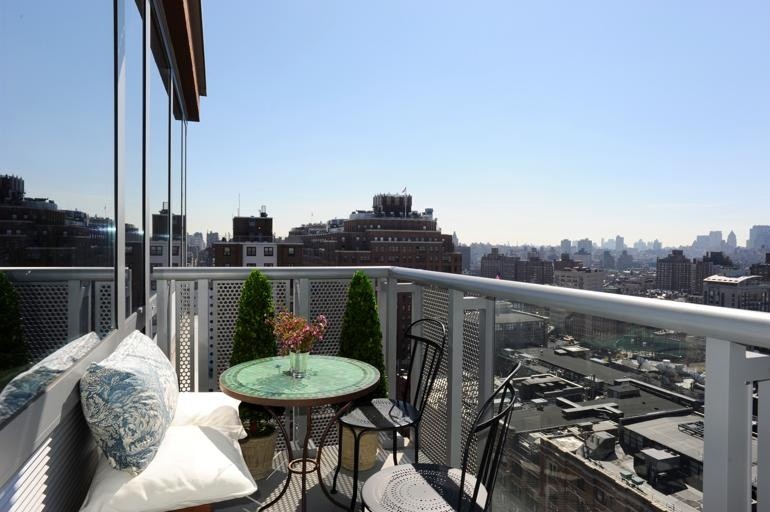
[264,310,328,357]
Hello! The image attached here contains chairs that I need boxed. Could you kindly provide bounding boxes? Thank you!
[330,315,522,512]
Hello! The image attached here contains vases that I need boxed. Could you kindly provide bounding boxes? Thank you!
[288,350,311,379]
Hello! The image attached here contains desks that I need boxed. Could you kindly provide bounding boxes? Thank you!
[219,353,381,512]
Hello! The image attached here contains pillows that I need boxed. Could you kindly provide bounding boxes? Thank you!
[78,328,258,511]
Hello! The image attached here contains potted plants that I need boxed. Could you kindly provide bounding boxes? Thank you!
[232,270,286,480]
[331,269,390,472]
